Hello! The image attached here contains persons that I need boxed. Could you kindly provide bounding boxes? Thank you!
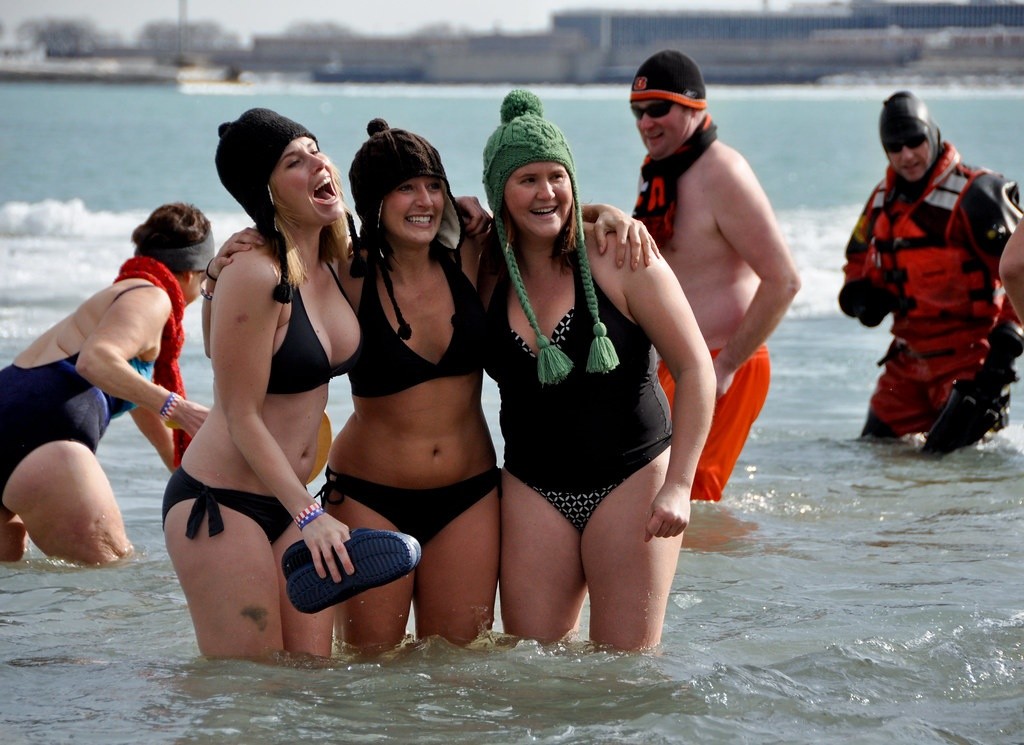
[630,47,801,503]
[1000,216,1022,320]
[195,117,668,662]
[0,204,219,563]
[475,89,719,667]
[838,90,1022,451]
[162,103,494,666]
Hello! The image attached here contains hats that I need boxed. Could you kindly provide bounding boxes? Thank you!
[216,106,323,302]
[879,91,941,170]
[351,119,467,345]
[629,49,707,110]
[483,90,621,387]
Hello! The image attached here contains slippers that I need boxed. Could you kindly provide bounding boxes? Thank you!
[282,527,422,616]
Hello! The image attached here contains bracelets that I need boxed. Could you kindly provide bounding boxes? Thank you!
[205,258,218,283]
[159,391,181,421]
[198,283,215,301]
[292,503,324,530]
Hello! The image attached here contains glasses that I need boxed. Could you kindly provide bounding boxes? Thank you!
[631,101,677,119]
[884,135,927,153]
